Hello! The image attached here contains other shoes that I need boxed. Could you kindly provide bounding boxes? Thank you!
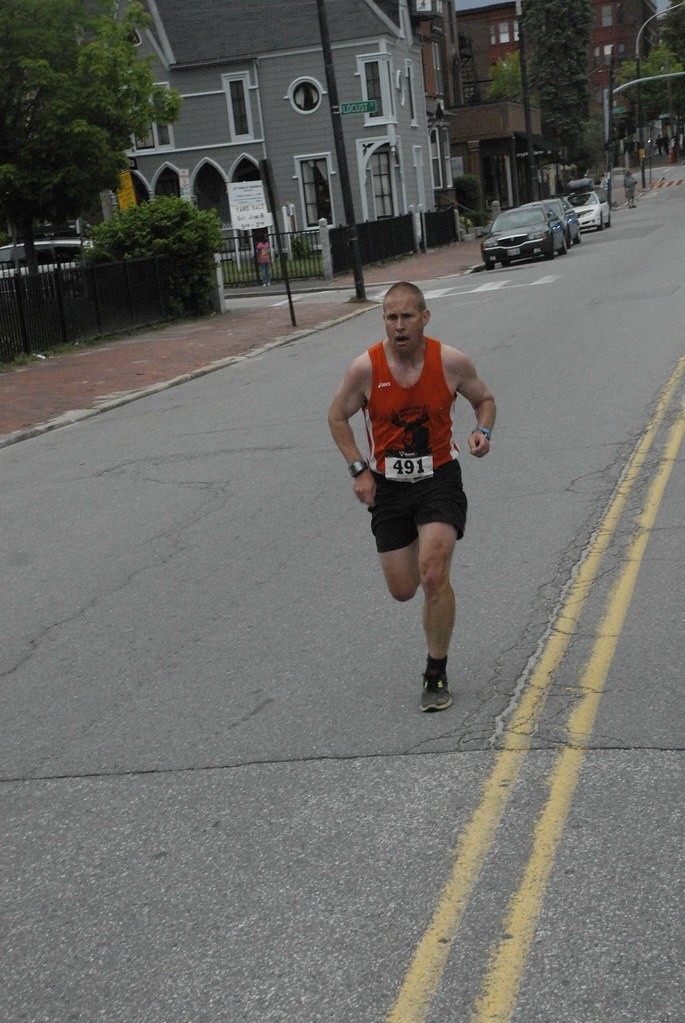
[263,284,266,287]
[267,283,271,286]
[632,205,636,208]
[629,206,632,208]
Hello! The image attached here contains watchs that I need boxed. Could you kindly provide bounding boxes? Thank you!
[472,427,491,441]
[347,460,368,477]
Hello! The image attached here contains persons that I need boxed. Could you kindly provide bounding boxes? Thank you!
[624,170,637,208]
[328,281,496,713]
[256,232,271,286]
[656,134,669,155]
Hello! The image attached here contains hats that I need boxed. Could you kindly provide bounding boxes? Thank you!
[625,171,632,177]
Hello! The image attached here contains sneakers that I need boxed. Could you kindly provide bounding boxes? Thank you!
[420,674,452,712]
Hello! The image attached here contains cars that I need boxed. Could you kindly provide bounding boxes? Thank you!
[481,203,568,270]
[520,198,581,250]
[0,239,95,294]
[563,178,611,231]
